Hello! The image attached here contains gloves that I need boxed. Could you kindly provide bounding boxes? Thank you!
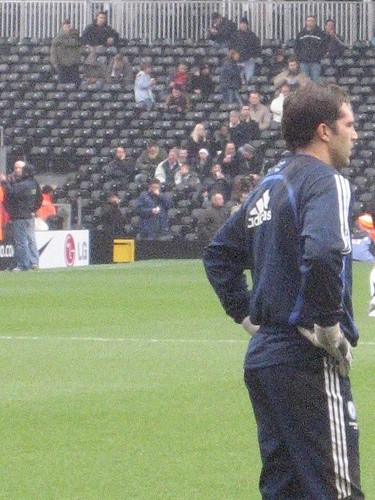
[240,314,262,337]
[315,323,354,368]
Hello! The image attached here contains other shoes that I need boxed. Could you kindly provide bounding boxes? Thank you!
[12,264,41,271]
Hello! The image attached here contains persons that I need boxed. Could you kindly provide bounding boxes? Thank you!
[0,10,375,317]
[203,81,365,500]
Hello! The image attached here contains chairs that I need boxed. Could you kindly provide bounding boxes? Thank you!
[1,37,375,243]
